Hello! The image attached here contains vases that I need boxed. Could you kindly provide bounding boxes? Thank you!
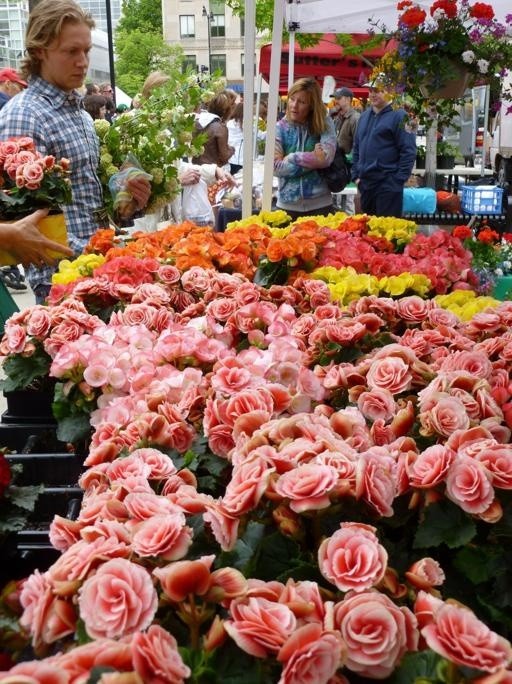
[417,62,471,97]
[1,210,69,267]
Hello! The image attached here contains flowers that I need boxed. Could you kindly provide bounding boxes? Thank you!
[0,135,75,220]
[91,64,229,223]
[351,0,512,135]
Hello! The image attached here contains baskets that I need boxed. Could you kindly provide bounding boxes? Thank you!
[461,185,504,216]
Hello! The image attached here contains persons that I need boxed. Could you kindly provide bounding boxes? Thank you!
[1,0,113,307]
[84,68,418,226]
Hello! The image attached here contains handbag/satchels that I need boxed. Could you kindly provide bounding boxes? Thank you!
[318,146,352,193]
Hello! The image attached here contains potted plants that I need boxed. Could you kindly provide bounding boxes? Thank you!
[416,139,461,169]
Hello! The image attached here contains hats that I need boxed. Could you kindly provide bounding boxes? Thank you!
[0,69,27,86]
[361,72,386,88]
[329,87,353,98]
